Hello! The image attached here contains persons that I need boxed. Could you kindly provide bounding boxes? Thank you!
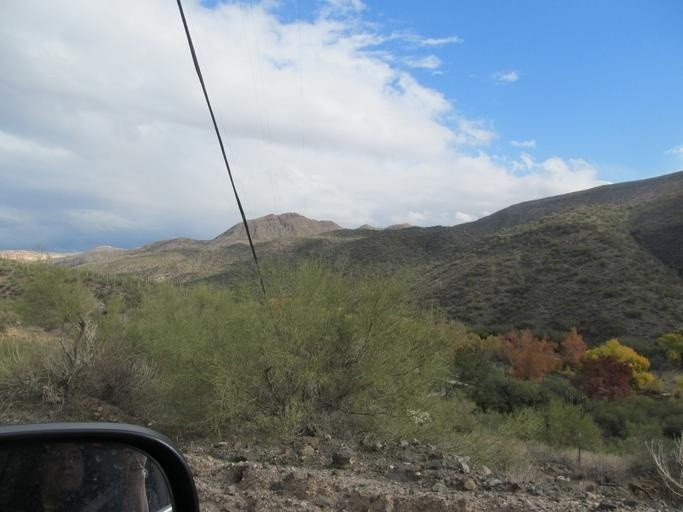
[0,441,174,512]
